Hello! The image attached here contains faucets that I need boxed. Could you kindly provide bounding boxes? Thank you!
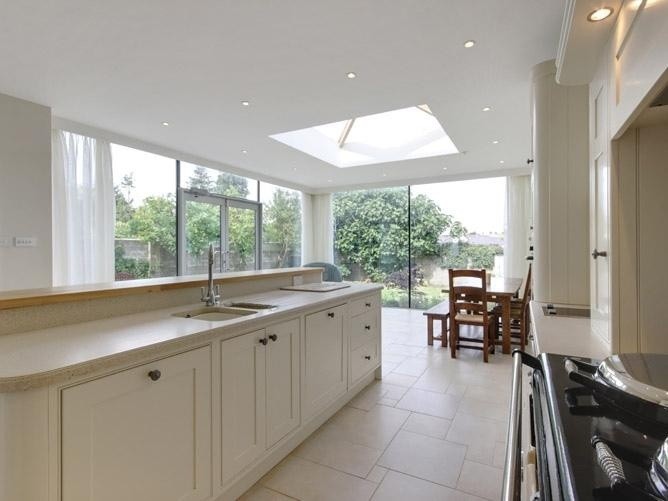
[201,244,219,305]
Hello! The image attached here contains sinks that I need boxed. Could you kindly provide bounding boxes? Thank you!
[170,307,256,321]
[225,302,276,309]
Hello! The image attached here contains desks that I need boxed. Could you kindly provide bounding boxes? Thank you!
[442,275,523,357]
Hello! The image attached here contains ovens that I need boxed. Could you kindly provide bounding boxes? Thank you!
[500,349,563,501]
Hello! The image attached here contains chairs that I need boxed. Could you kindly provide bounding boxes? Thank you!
[449,267,496,362]
[493,262,532,352]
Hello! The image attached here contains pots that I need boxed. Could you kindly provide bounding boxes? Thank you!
[590,435,668,501]
[564,352,668,428]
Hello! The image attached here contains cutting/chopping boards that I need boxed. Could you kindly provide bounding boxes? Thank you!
[280,281,351,292]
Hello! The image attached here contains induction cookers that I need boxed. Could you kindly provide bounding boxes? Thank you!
[540,351,668,501]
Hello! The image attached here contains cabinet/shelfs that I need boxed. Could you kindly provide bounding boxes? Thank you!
[590,72,667,359]
[219,317,302,501]
[61,344,212,499]
[305,304,346,435]
[349,292,383,386]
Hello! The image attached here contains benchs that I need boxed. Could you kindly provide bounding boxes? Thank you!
[423,293,450,347]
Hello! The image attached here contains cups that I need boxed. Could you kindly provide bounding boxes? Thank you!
[486,273,491,285]
